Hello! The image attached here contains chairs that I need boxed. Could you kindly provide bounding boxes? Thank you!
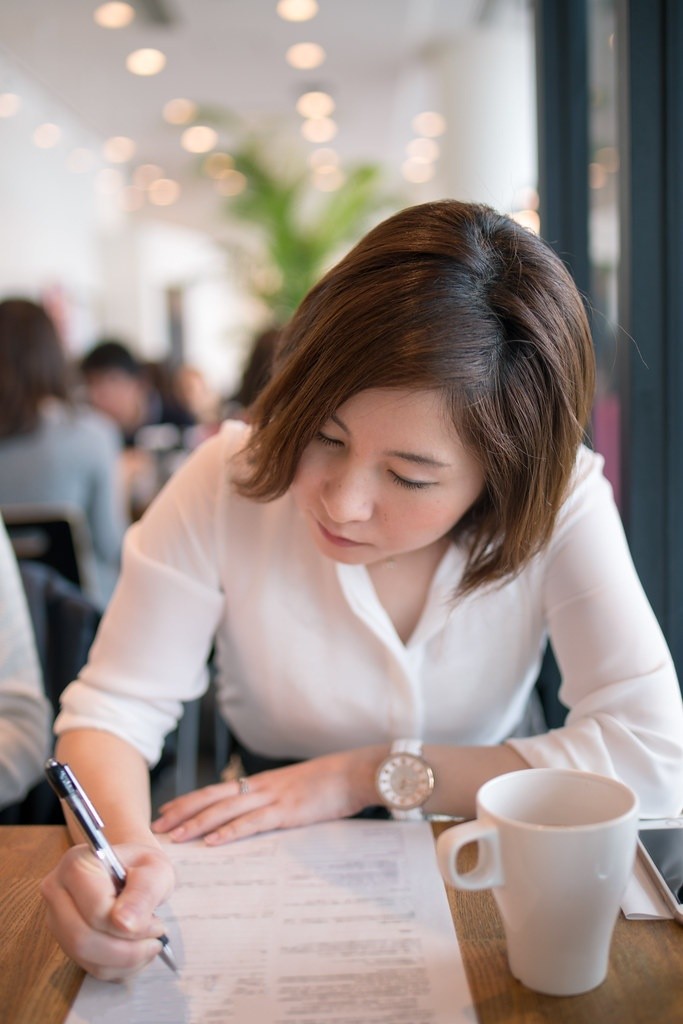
[6,506,86,596]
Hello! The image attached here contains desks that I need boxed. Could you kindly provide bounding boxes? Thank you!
[0,824,683,1024]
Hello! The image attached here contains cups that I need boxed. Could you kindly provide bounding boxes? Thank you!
[436,768,643,997]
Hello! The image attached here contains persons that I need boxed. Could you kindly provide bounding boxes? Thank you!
[1,299,131,613]
[208,321,280,444]
[38,200,683,983]
[80,340,197,514]
[0,521,55,807]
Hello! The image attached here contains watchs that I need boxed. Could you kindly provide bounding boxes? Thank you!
[375,734,438,821]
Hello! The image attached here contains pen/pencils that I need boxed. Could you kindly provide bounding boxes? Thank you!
[41,754,188,984]
